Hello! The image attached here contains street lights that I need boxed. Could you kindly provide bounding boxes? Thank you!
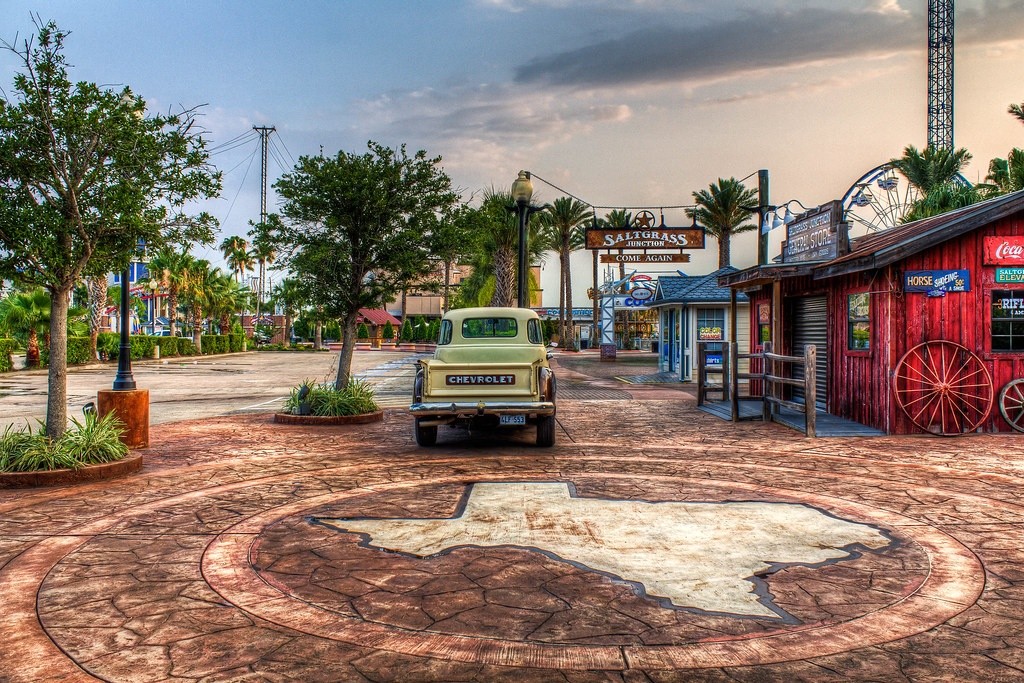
[95,96,155,450]
[147,277,162,360]
[511,170,532,310]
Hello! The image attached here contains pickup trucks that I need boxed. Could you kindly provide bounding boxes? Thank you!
[406,307,560,445]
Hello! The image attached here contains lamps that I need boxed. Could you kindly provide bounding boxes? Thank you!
[772,206,807,230]
[783,200,820,227]
[762,211,797,235]
